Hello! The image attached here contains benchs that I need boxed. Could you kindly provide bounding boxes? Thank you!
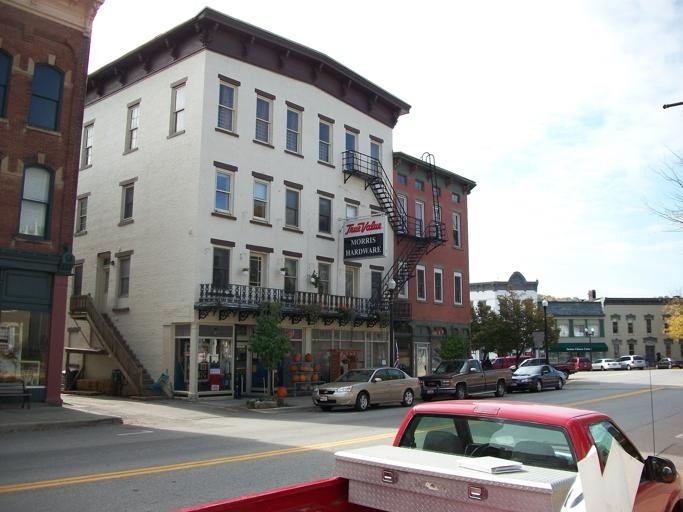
[0,378,34,410]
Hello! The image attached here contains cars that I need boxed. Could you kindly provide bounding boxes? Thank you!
[253,365,308,388]
[654,357,682,369]
[590,358,621,371]
[311,366,421,411]
[489,355,592,381]
[0,344,51,382]
[506,364,566,394]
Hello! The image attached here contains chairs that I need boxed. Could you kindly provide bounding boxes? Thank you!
[420,429,467,455]
[509,439,577,474]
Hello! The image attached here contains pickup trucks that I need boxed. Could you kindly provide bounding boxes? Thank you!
[164,399,682,511]
[417,358,512,401]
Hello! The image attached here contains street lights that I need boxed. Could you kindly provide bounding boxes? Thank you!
[584,327,594,361]
[541,298,548,365]
[387,278,396,367]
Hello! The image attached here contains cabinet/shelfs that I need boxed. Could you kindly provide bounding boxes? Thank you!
[285,359,326,398]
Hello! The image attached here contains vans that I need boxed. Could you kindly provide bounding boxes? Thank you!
[613,355,646,370]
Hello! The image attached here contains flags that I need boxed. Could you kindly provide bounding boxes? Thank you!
[394,341,399,369]
